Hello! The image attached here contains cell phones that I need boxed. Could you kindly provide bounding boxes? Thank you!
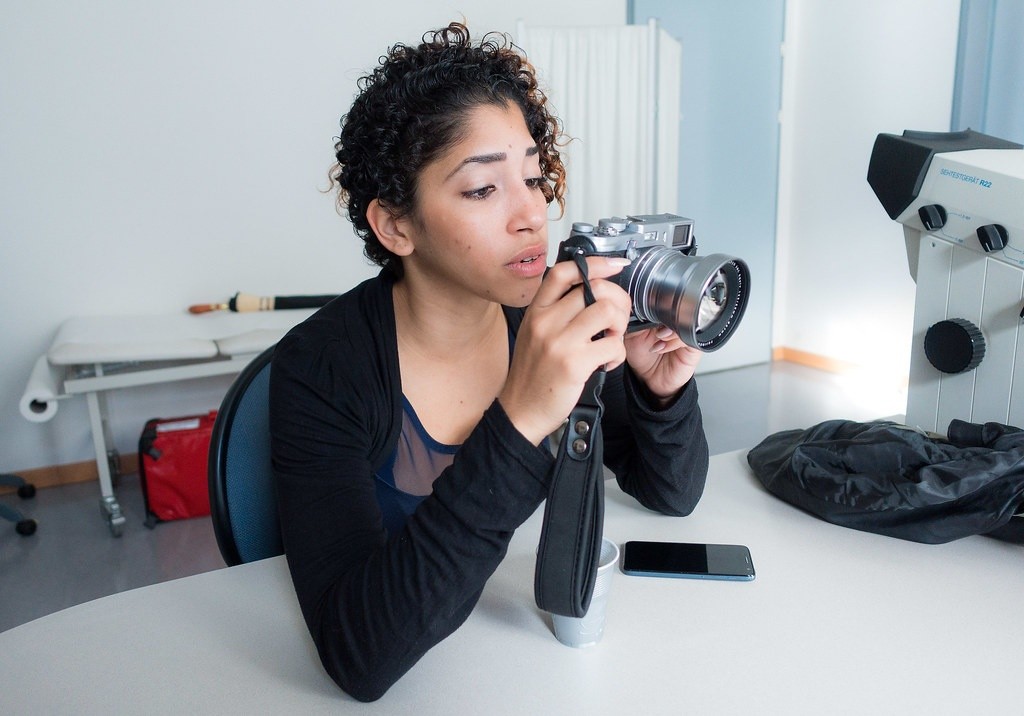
[621,541,755,581]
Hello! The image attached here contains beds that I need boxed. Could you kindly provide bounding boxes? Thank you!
[19,306,326,537]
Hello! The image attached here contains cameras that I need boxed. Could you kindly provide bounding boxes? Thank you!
[556,213,751,353]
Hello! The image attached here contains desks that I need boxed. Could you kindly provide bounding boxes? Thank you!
[0,446,1024,715]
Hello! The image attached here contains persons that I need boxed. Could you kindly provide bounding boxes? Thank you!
[270,23,708,703]
